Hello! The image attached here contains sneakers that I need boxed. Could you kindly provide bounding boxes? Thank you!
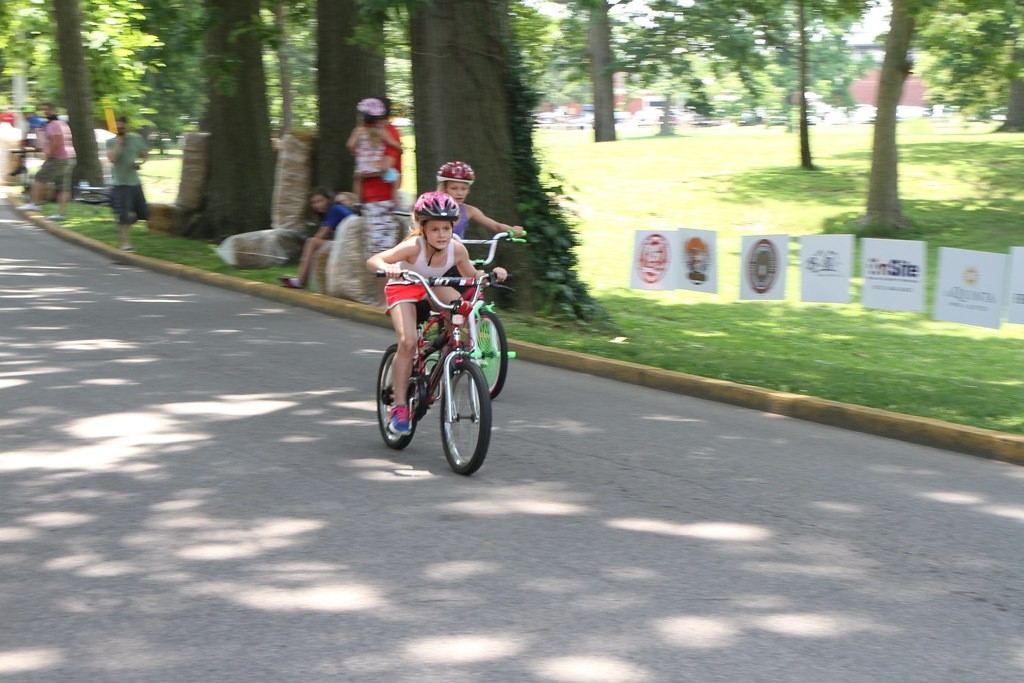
[388,404,409,436]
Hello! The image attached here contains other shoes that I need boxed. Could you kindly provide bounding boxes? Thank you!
[10,167,28,176]
[48,214,65,220]
[282,277,305,288]
[390,206,411,216]
[16,203,43,212]
[123,247,135,254]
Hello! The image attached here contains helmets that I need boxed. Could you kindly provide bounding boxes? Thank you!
[356,98,387,117]
[414,192,460,222]
[436,161,476,185]
[21,103,36,114]
[0,114,13,124]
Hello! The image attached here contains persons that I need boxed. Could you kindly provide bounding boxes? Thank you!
[277,96,404,314]
[0,102,78,222]
[105,116,149,251]
[409,160,524,264]
[366,191,509,438]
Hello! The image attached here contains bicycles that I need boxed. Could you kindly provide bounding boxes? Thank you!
[415,229,528,399]
[375,269,518,477]
